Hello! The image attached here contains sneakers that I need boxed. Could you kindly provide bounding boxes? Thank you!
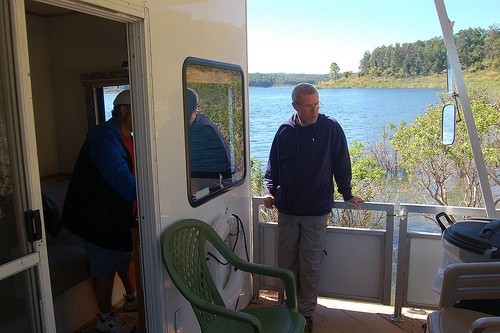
[304,316,313,333]
[123,294,138,310]
[96,309,136,333]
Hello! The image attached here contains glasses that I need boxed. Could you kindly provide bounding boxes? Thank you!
[296,102,322,110]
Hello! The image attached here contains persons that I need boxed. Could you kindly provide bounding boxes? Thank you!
[264,84,364,333]
[62,90,136,333]
[188,87,234,193]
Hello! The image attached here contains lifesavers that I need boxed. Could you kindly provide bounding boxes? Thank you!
[203,215,247,309]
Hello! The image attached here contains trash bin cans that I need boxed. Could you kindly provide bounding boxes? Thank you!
[432,211,500,316]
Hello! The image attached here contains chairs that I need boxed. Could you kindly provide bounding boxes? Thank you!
[425,261,500,333]
[163,219,310,333]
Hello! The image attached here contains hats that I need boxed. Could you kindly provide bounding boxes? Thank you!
[113,90,130,104]
